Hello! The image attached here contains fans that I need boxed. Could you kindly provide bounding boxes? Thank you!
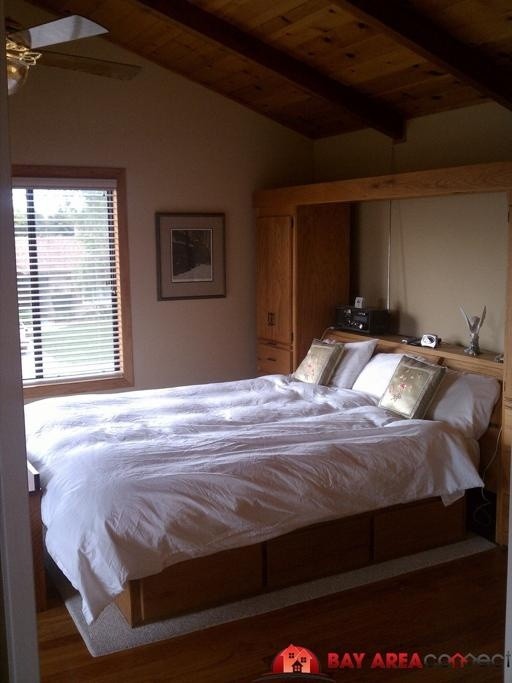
[3,13,142,81]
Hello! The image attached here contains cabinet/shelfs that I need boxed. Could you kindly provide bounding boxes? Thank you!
[255,214,292,376]
[495,404,512,545]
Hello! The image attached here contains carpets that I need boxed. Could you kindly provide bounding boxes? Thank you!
[46,530,496,656]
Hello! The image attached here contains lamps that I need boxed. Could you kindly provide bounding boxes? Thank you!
[4,37,42,101]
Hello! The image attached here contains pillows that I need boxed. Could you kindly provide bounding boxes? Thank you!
[331,339,380,389]
[294,339,345,387]
[352,352,425,401]
[425,368,501,441]
[378,355,449,420]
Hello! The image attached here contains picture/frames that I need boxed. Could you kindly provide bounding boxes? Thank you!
[154,210,227,299]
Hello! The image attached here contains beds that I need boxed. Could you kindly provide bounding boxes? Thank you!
[23,327,501,628]
